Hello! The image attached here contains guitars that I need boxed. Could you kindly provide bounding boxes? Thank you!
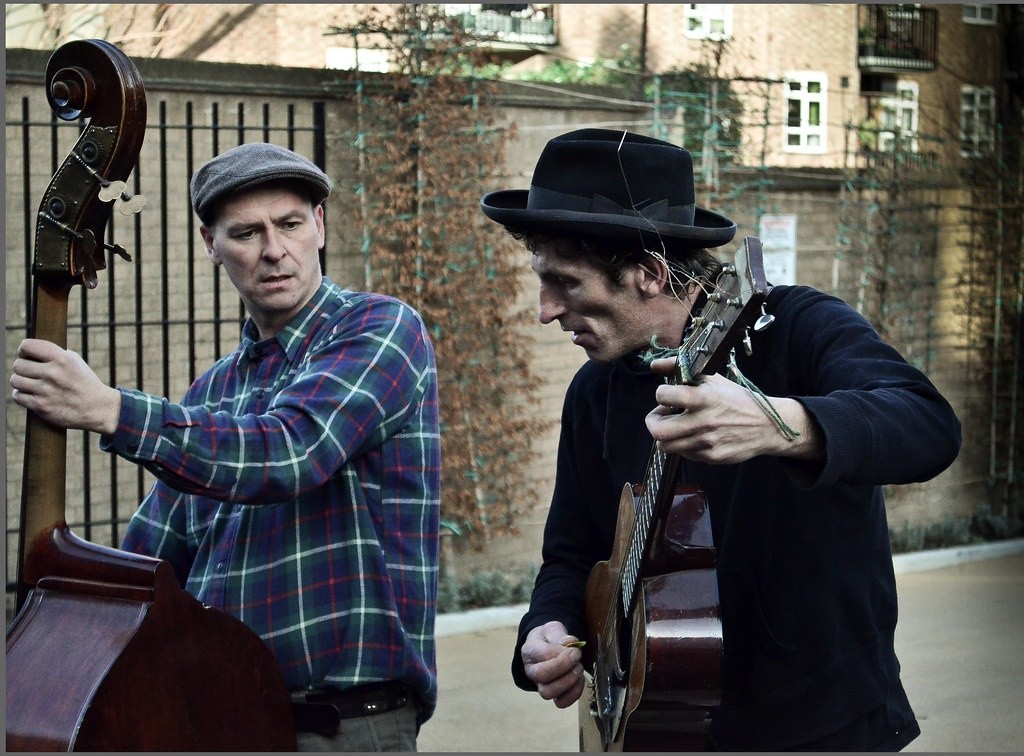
[574,234,770,754]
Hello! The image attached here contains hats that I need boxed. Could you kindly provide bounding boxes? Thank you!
[190,144,330,223]
[480,129,736,247]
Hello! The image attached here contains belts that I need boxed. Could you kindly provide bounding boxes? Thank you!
[291,675,412,736]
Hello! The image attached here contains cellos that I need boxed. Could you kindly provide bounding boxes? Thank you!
[1,35,310,756]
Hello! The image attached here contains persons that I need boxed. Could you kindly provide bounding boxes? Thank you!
[10,141,442,755]
[480,127,962,752]
